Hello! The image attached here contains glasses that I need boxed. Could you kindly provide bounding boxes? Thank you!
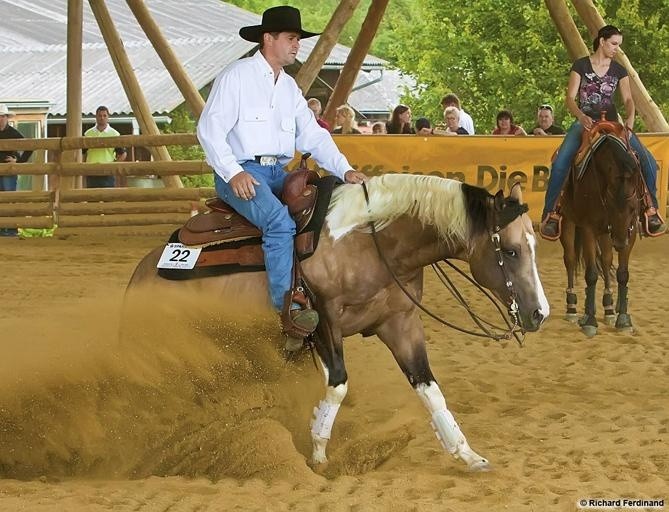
[540,105,552,110]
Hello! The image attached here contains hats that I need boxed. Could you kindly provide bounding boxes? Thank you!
[0,103,16,118]
[239,6,324,42]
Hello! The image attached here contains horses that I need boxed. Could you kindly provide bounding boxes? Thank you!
[558,127,646,339]
[123,169,551,478]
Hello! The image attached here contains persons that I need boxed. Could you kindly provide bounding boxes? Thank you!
[538,26,667,240]
[195,6,370,357]
[82,106,128,216]
[1,103,33,236]
[307,94,565,136]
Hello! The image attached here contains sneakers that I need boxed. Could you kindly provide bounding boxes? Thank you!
[641,215,665,234]
[281,310,320,351]
[544,218,558,235]
